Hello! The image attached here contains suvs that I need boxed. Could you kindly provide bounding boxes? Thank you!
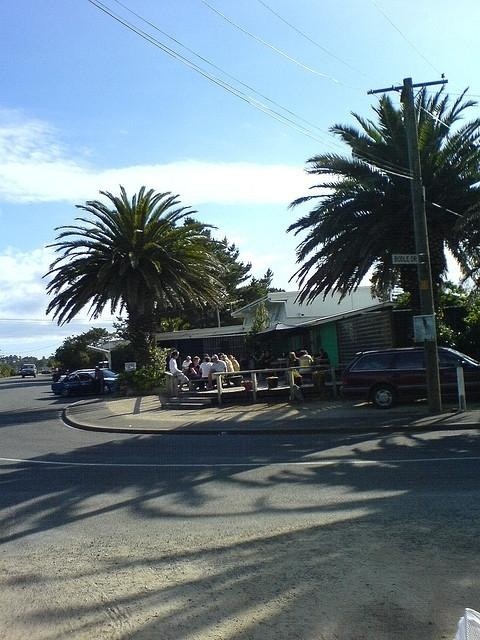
[21,363,38,379]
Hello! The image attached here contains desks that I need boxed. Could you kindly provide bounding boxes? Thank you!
[270,360,290,378]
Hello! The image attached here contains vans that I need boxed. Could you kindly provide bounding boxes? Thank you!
[340,347,479,409]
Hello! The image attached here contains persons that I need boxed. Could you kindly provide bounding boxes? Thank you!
[263,348,329,385]
[169,350,240,391]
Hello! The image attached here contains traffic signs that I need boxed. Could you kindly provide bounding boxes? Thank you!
[392,252,422,265]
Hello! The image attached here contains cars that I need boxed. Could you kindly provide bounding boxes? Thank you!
[41,369,51,375]
[50,367,122,399]
[18,367,22,374]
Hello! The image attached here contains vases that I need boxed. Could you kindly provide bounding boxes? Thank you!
[266,377,278,388]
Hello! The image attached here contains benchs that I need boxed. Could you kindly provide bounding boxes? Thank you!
[188,374,244,390]
